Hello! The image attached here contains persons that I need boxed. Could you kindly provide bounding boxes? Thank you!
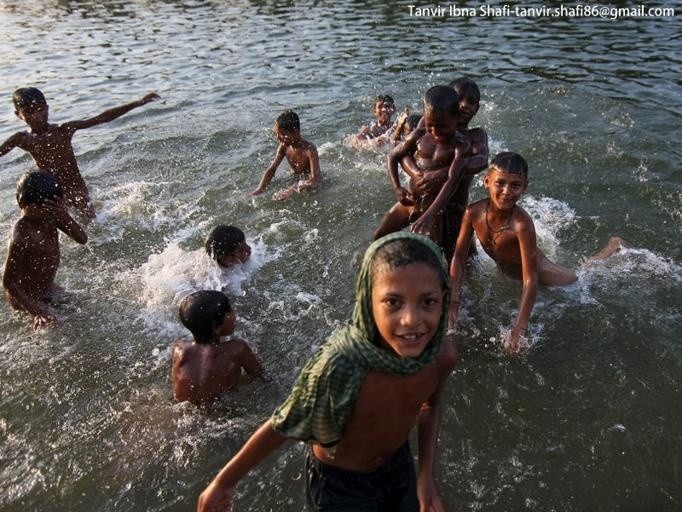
[171,290,264,407]
[204,224,251,268]
[448,152,623,353]
[358,95,422,146]
[0,88,162,330]
[197,231,457,512]
[374,77,489,261]
[250,111,321,200]
